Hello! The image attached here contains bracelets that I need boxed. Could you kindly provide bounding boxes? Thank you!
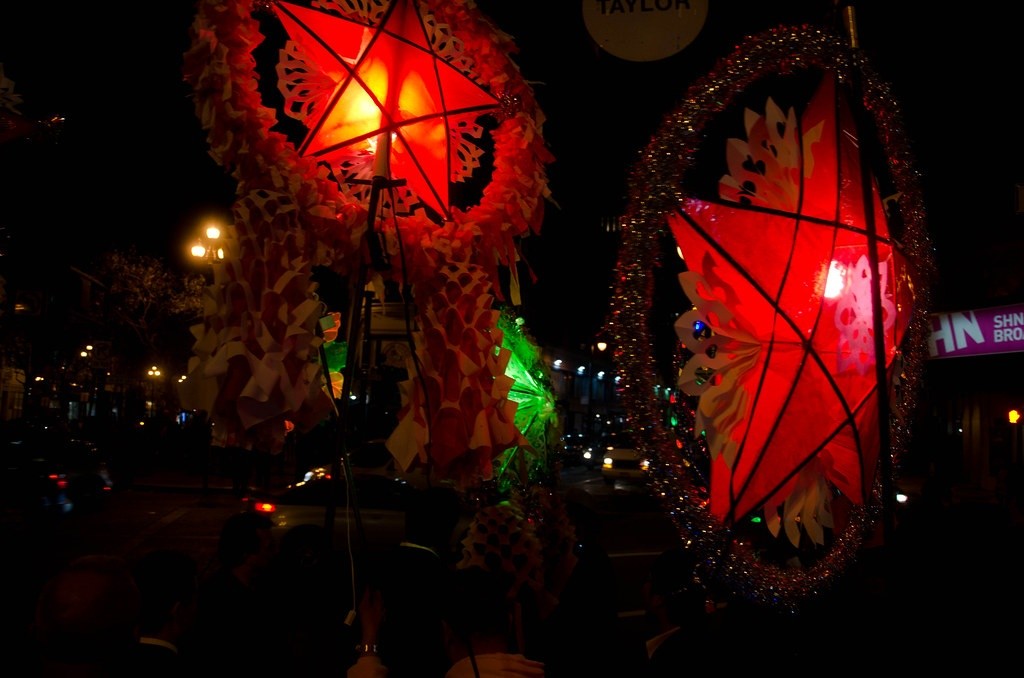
[356,641,378,654]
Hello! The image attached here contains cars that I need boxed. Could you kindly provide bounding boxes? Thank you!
[555,423,650,487]
[244,441,412,570]
[1,427,115,519]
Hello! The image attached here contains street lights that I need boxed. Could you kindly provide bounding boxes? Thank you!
[588,335,607,415]
[149,364,161,414]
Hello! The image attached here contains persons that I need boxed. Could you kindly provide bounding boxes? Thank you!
[1,459,1024,678]
